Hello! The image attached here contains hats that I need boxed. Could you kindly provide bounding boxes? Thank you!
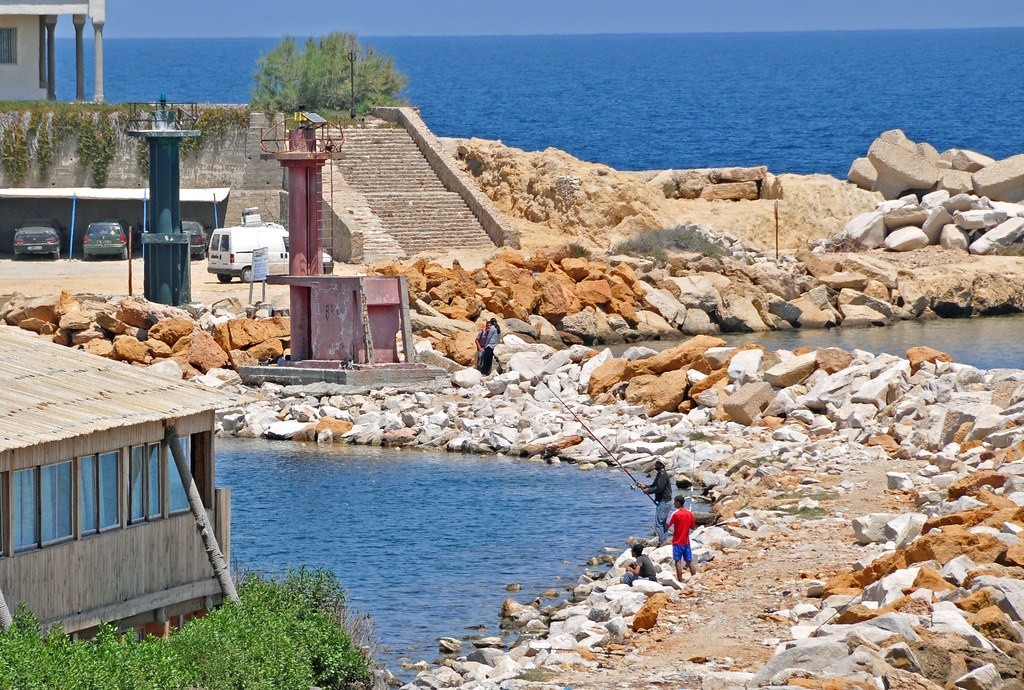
[656,454,668,466]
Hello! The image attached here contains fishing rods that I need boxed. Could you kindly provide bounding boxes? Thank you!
[665,418,700,536]
[528,367,659,507]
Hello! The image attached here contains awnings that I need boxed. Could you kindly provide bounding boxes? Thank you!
[0,188,232,262]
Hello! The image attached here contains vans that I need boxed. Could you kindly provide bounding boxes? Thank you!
[206,226,334,283]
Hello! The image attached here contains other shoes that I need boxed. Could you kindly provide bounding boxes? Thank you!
[657,541,666,548]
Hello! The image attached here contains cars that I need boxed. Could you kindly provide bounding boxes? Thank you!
[181,221,212,260]
[13,217,68,259]
[83,220,127,260]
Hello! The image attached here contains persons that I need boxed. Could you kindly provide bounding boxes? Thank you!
[623,544,658,586]
[665,496,696,581]
[475,321,492,370]
[636,456,673,547]
[481,318,500,374]
